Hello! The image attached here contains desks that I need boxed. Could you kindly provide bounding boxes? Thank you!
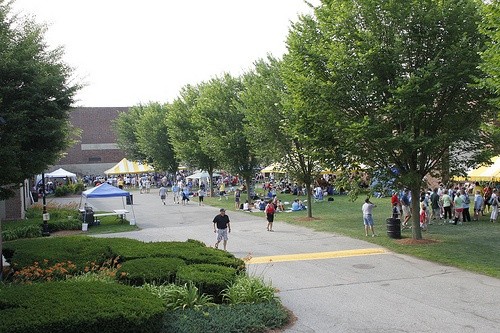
[93,209,129,223]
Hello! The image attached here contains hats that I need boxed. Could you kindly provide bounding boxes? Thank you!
[220,209,225,212]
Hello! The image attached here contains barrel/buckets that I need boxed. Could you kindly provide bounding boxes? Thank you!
[385,218,402,239]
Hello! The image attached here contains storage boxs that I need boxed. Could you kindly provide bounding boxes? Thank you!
[94,220,100,225]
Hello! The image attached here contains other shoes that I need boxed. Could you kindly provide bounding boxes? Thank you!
[402,223,406,227]
[372,234,379,237]
[420,214,486,231]
[366,234,371,236]
[215,243,218,248]
[266,227,269,231]
[269,230,274,232]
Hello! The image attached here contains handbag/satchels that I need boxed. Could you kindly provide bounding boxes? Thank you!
[485,197,497,206]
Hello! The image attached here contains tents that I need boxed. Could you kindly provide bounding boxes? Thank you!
[186,171,221,179]
[104,158,154,175]
[260,162,288,173]
[452,154,500,182]
[79,183,136,225]
[45,168,76,178]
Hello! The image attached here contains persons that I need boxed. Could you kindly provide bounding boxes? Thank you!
[391,182,500,232]
[31,176,77,198]
[159,183,168,205]
[265,199,275,232]
[82,173,169,194]
[240,171,348,212]
[213,209,231,251]
[169,170,246,208]
[362,198,379,237]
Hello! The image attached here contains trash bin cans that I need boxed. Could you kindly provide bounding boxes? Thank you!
[386,218,401,239]
